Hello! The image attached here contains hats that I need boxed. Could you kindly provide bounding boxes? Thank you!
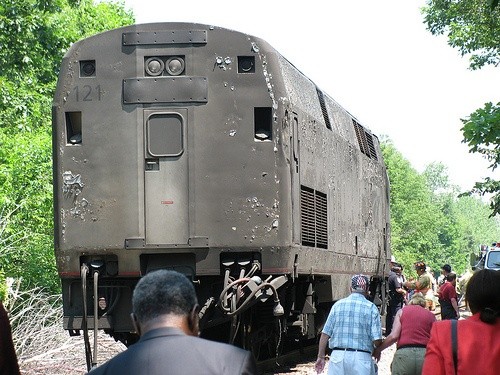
[350,273,370,291]
[414,262,427,270]
[391,255,396,263]
[441,265,452,272]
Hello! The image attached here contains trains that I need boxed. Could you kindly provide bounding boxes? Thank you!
[52,22,391,342]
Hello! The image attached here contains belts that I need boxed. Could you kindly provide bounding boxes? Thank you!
[397,344,426,350]
[332,347,371,353]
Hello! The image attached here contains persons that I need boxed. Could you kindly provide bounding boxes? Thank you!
[314,254,500,375]
[84,270,258,375]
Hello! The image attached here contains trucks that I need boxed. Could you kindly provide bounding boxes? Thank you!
[471,243,500,271]
